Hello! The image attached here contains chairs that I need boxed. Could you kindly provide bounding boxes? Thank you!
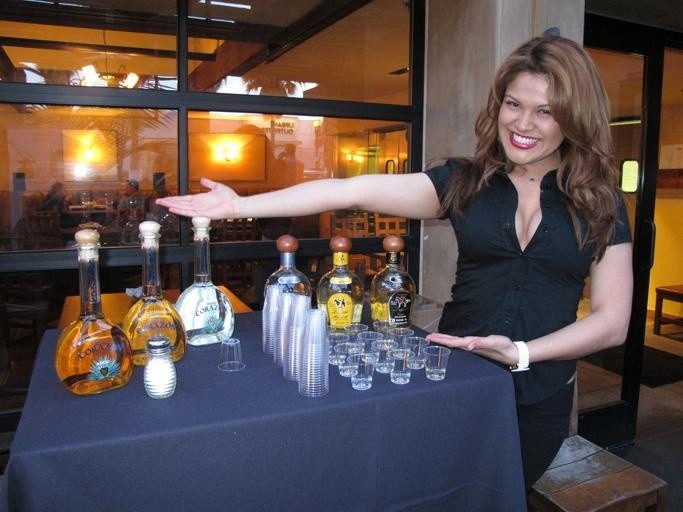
[374,214,407,269]
[218,217,255,288]
[29,214,61,249]
[331,212,371,272]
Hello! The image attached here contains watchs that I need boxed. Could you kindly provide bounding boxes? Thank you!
[509,341,530,373]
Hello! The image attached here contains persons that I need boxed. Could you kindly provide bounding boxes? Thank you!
[40,182,76,228]
[155,35,634,499]
[118,178,146,243]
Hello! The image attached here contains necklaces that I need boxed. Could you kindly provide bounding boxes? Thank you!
[516,167,555,182]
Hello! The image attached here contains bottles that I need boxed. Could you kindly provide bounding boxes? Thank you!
[368,234,417,338]
[143,336,177,399]
[175,215,234,346]
[54,229,134,396]
[263,236,312,298]
[121,222,187,366]
[74,191,143,246]
[316,236,363,327]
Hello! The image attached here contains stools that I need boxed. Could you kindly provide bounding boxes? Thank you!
[528,435,668,512]
[654,284,683,340]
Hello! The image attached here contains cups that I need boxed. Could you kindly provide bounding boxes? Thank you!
[261,284,329,398]
[217,341,245,371]
[326,319,452,391]
